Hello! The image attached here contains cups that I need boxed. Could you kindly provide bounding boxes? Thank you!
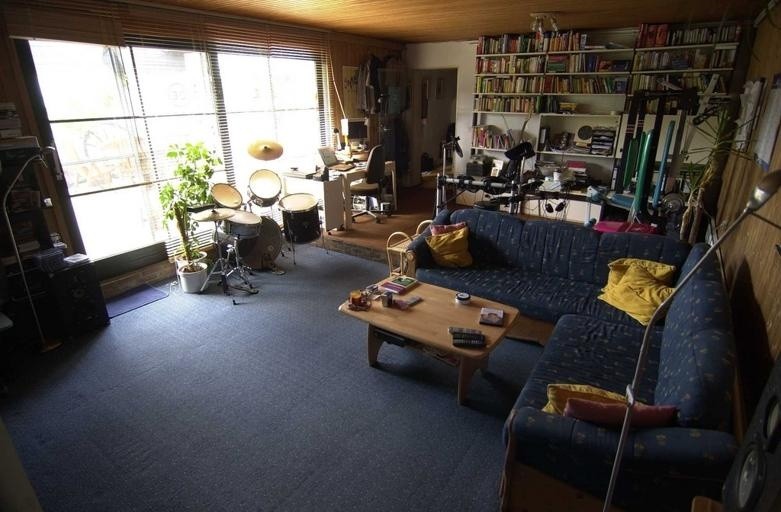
[351,290,361,305]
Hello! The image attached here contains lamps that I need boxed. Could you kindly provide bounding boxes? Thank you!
[603,166,781,511]
[2,146,64,354]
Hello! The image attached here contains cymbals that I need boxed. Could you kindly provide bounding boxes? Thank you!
[193,208,234,222]
[248,140,283,160]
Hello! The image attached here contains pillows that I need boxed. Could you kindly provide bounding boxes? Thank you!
[597,263,677,326]
[430,222,466,236]
[600,258,677,293]
[564,397,680,433]
[541,383,649,417]
[469,236,508,271]
[424,227,473,268]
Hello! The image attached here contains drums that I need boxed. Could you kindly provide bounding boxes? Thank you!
[224,209,262,239]
[279,192,321,244]
[210,183,243,210]
[213,216,283,269]
[248,169,282,207]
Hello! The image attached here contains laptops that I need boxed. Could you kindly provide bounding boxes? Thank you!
[319,147,355,171]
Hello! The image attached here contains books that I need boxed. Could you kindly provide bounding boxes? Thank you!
[630,48,735,72]
[566,160,589,190]
[471,124,516,150]
[585,44,606,49]
[380,276,418,295]
[476,29,587,54]
[478,54,612,73]
[63,253,89,265]
[479,307,503,327]
[476,77,626,95]
[472,95,559,115]
[609,41,630,48]
[631,74,727,115]
[637,22,741,48]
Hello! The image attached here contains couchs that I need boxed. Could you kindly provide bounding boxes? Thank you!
[405,207,691,346]
[499,242,746,511]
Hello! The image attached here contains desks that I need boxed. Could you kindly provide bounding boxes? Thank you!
[317,160,397,230]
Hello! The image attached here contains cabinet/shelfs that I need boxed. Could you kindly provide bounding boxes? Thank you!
[606,19,754,214]
[283,172,344,235]
[470,27,640,225]
[387,220,433,275]
[0,136,55,342]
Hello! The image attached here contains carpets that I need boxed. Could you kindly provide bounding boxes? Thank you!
[105,283,169,319]
[1,241,554,512]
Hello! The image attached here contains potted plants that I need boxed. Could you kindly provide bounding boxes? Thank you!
[166,143,223,268]
[159,182,209,293]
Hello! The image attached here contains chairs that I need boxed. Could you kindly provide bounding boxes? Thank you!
[350,144,393,223]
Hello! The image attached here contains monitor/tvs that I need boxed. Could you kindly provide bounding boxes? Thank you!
[341,118,370,148]
